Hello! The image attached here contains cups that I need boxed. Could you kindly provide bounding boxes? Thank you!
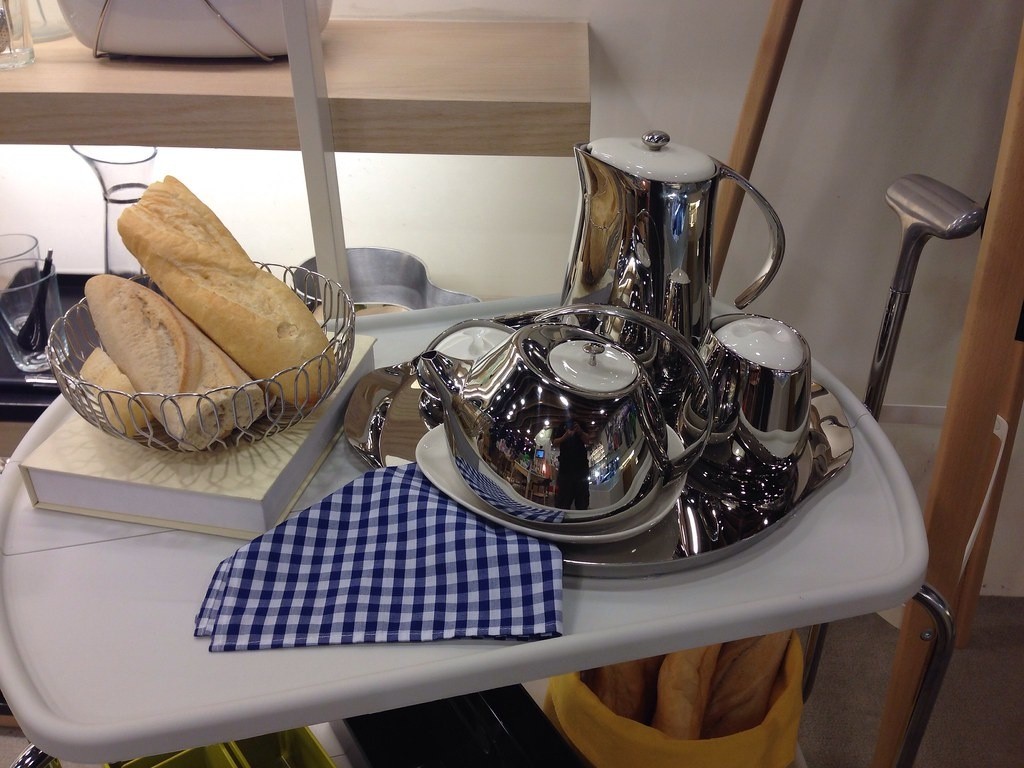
[0,233,42,336]
[675,312,817,554]
[0,0,37,72]
[0,255,69,372]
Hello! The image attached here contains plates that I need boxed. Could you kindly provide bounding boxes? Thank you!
[413,417,690,549]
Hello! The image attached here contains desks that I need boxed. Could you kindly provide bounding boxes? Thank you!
[0,292,931,768]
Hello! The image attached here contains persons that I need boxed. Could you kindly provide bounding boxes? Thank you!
[648,179,667,323]
[751,365,775,434]
[549,407,595,510]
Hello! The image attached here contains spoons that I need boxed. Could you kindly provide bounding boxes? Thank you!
[17,247,54,350]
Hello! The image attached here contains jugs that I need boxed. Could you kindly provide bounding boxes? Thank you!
[556,132,788,405]
[420,303,715,534]
[71,132,182,294]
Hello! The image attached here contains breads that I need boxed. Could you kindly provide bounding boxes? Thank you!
[82,175,337,452]
[580,629,795,741]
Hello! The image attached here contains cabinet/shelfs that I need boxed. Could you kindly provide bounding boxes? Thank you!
[0,2,599,722]
